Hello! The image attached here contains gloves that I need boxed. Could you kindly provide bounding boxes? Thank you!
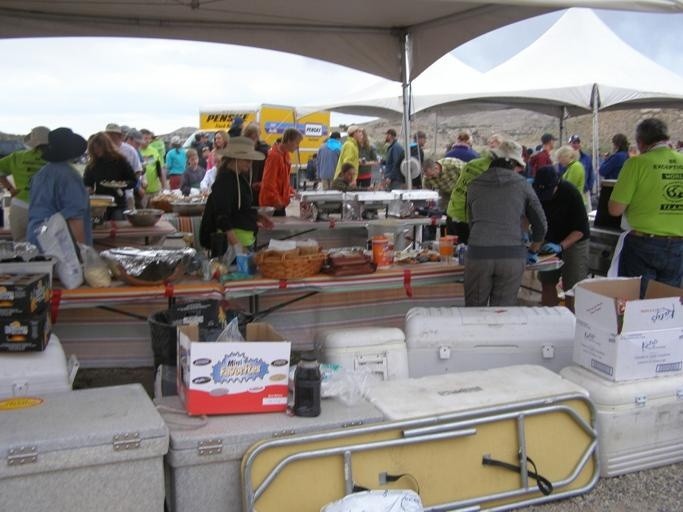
[526,250,538,265]
[521,230,529,243]
[542,243,563,254]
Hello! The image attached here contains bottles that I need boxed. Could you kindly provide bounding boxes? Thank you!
[292,352,321,417]
[439,234,453,265]
[370,235,392,270]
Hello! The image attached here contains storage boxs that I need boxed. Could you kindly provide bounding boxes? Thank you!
[1,332,79,395]
[1,254,57,287]
[174,323,290,414]
[560,362,683,478]
[151,378,385,512]
[1,273,50,318]
[573,278,683,381]
[365,363,589,420]
[406,305,576,378]
[311,324,408,379]
[0,382,169,511]
[0,302,54,351]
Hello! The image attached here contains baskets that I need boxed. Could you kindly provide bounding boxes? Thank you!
[254,248,327,277]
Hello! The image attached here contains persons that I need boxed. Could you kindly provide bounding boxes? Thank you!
[526,148,533,161]
[447,141,526,244]
[558,135,592,212]
[593,134,630,229]
[139,129,166,209]
[522,144,527,168]
[423,157,466,207]
[125,128,149,210]
[444,133,480,163]
[190,134,210,170]
[200,150,226,197]
[446,134,504,243]
[200,137,275,264]
[333,163,358,191]
[395,131,426,189]
[228,113,270,207]
[526,152,547,186]
[83,132,138,221]
[316,131,343,190]
[208,129,230,171]
[675,140,683,151]
[532,165,590,313]
[381,129,404,191]
[181,149,206,189]
[463,157,548,306]
[540,133,558,165]
[0,126,50,256]
[165,136,187,191]
[355,128,380,191]
[27,128,92,263]
[200,132,213,152]
[333,126,360,189]
[608,118,683,299]
[307,153,317,181]
[104,124,143,210]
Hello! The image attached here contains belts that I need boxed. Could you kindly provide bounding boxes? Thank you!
[631,230,683,241]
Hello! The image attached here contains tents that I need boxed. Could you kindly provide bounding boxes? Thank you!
[407,7,683,197]
[292,50,487,192]
[0,1,683,189]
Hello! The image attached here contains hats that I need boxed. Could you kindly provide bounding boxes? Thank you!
[348,126,358,135]
[232,117,245,128]
[200,132,210,138]
[331,133,340,138]
[106,124,122,134]
[24,127,50,147]
[171,136,181,144]
[541,134,557,142]
[121,126,130,133]
[41,129,88,162]
[491,141,526,168]
[568,135,580,143]
[222,136,265,162]
[129,129,145,144]
[382,129,397,137]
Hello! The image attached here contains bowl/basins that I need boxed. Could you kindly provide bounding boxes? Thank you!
[258,207,275,216]
[101,247,196,286]
[171,203,204,216]
[124,209,164,226]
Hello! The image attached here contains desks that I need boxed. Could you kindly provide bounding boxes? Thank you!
[239,393,600,511]
